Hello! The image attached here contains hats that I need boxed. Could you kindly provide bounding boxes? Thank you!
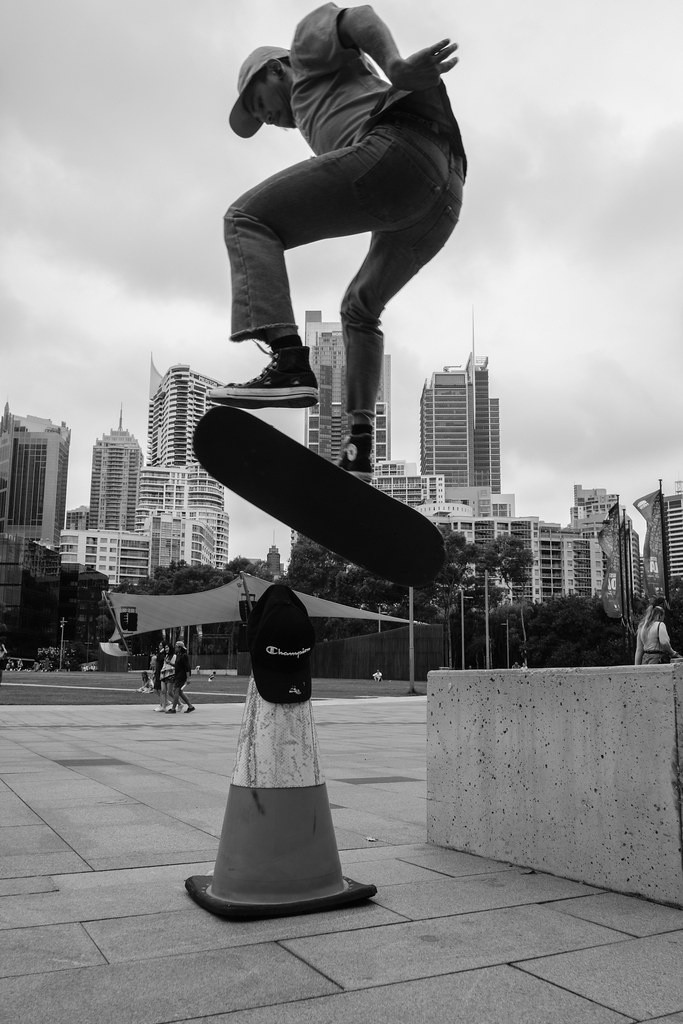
[248,584,316,704]
[175,641,187,651]
[229,46,291,139]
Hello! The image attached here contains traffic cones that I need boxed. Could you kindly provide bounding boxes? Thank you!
[183,668,380,923]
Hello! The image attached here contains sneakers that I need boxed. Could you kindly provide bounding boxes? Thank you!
[208,338,319,410]
[338,423,373,483]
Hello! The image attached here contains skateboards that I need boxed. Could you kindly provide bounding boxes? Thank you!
[191,406,447,590]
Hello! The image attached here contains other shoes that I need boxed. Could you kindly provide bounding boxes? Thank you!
[184,706,195,713]
[156,707,166,712]
[165,709,176,714]
[176,703,184,712]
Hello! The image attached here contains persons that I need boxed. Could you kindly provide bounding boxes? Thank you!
[154,641,195,714]
[206,1,468,485]
[635,605,679,665]
[208,671,216,689]
[373,670,382,682]
[141,672,154,691]
[0,644,7,686]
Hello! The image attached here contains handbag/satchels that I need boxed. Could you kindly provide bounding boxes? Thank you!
[658,654,670,664]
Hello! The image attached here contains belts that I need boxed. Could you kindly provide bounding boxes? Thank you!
[643,651,663,654]
